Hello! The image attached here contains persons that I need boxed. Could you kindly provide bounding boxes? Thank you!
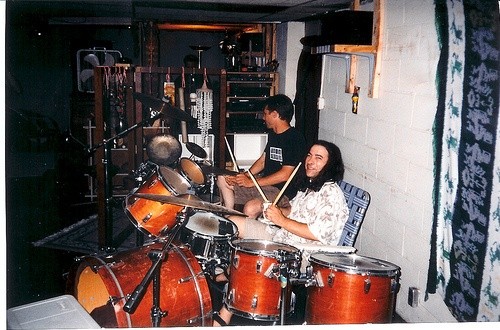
[216,93,307,218]
[174,53,206,92]
[212,140,349,327]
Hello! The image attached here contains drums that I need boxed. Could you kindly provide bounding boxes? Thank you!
[120,165,196,242]
[170,157,206,190]
[224,239,302,321]
[64,238,214,327]
[174,210,238,277]
[302,252,401,324]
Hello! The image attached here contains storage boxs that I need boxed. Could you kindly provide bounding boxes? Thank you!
[7,295,101,330]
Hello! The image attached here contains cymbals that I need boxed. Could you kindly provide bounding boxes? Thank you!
[132,91,196,123]
[128,192,246,215]
[146,134,182,165]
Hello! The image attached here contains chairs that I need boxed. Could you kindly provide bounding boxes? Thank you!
[293,181,371,253]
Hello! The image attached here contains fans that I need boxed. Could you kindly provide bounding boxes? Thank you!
[77,49,123,93]
